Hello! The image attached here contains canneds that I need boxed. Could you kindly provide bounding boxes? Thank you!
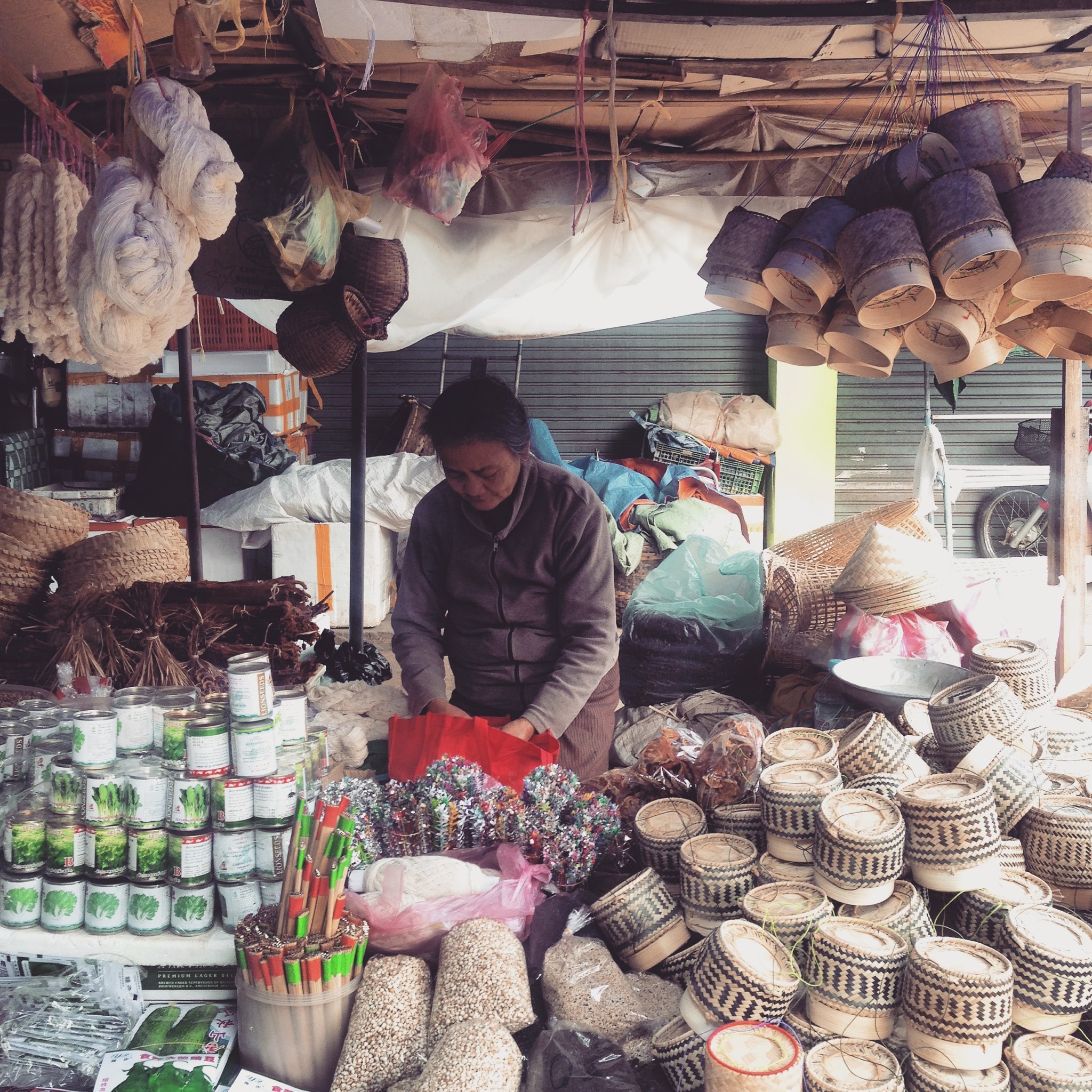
[0,648,330,936]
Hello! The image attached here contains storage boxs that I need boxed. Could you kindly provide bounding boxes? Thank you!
[1,294,395,631]
[636,423,765,496]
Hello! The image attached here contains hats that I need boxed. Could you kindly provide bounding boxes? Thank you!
[831,522,958,616]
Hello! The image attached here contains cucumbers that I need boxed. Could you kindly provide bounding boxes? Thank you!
[125,1004,220,1057]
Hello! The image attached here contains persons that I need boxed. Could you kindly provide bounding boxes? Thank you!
[391,375,621,777]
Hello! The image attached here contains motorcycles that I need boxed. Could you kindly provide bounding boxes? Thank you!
[976,369,1092,559]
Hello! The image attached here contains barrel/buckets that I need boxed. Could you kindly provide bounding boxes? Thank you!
[235,964,364,1092]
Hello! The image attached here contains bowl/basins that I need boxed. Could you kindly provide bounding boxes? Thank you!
[831,655,970,715]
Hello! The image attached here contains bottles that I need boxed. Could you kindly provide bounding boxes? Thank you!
[813,659,853,732]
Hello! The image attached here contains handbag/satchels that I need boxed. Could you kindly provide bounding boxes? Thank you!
[387,712,560,793]
[345,842,553,956]
[617,534,762,704]
[249,96,372,291]
[381,65,510,226]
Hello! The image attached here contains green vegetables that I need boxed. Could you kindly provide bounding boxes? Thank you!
[111,1060,218,1092]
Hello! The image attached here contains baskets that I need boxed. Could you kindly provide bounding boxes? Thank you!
[370,394,435,456]
[652,443,764,496]
[1014,418,1051,464]
[169,294,278,350]
[0,486,188,658]
